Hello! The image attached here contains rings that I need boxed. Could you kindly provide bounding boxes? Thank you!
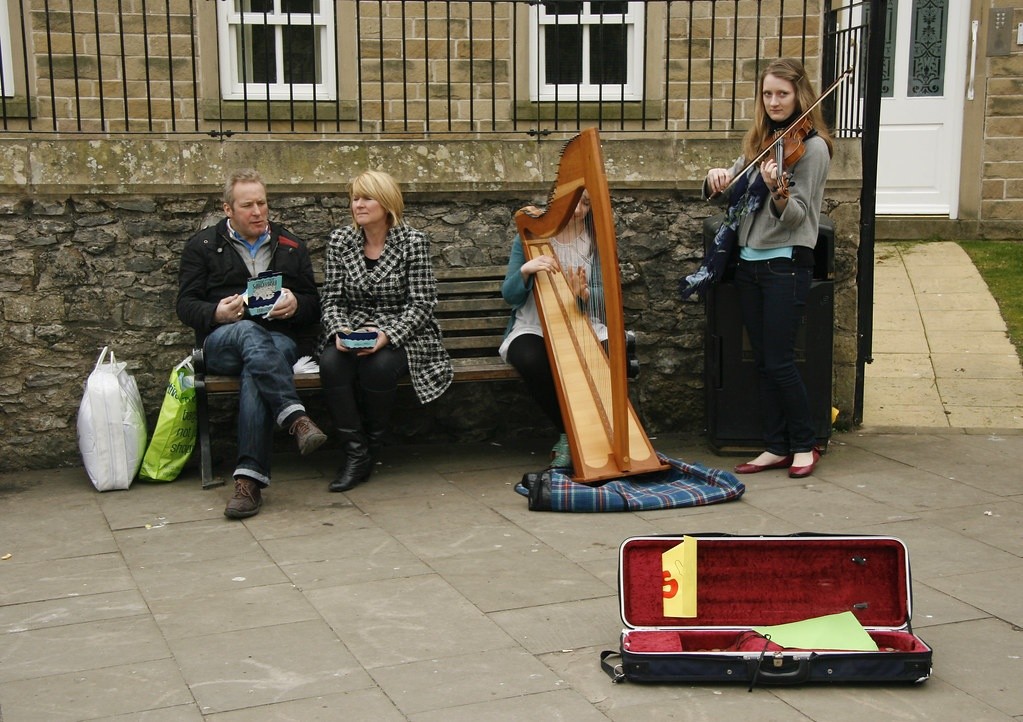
[284,312,289,318]
[237,312,242,317]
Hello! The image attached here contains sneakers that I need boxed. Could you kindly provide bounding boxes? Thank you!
[289,416,328,455]
[224,479,263,517]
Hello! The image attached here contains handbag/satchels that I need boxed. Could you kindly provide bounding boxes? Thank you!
[514,450,746,512]
[76,346,147,492]
[138,354,197,483]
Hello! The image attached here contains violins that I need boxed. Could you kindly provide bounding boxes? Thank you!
[760,115,813,201]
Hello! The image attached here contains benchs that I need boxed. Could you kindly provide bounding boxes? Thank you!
[193,266,640,490]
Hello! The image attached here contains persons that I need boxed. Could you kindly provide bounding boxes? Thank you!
[176,167,328,519]
[320,168,455,491]
[700,56,833,479]
[499,176,610,477]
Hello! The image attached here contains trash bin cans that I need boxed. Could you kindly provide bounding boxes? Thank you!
[701,212,837,452]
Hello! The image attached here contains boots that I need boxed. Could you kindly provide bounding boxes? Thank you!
[334,378,399,474]
[548,433,572,468]
[323,379,373,491]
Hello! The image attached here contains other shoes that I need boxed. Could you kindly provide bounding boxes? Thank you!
[734,455,793,473]
[789,449,820,478]
